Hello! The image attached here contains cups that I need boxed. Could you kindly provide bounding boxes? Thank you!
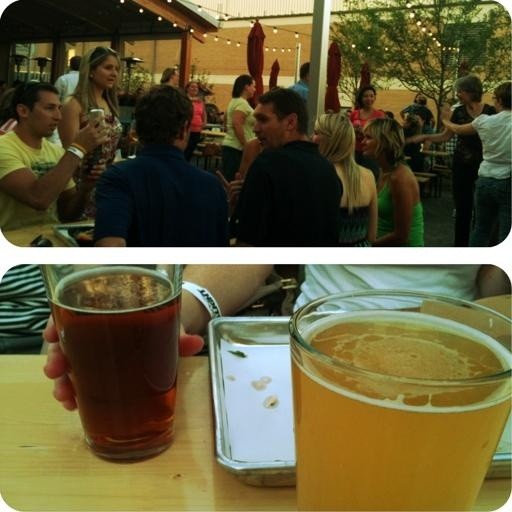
[288,291,511,507]
[89,106,107,130]
[37,263,184,463]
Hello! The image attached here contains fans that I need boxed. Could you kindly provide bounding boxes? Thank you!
[0,350,511,510]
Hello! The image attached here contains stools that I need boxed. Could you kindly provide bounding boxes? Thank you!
[411,171,439,202]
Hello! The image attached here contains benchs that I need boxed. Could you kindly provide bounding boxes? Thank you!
[193,141,221,170]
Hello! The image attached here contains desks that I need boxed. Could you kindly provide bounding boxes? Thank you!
[201,130,227,171]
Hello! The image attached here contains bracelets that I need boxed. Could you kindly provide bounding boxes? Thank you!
[179,280,223,323]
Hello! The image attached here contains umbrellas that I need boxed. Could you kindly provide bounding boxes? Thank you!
[324,40,342,113]
[246,18,267,109]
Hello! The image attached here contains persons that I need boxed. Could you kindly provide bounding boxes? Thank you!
[0,45,512,246]
[42,264,512,412]
